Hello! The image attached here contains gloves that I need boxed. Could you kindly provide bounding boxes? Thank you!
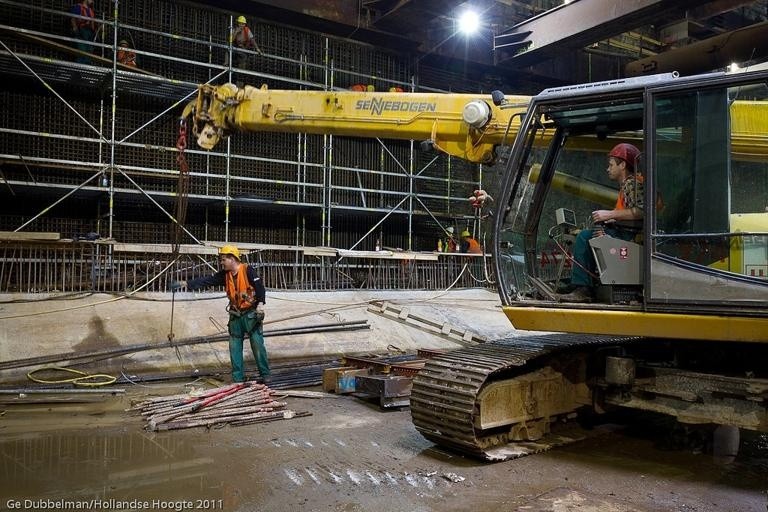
[168,280,182,289]
[256,304,266,319]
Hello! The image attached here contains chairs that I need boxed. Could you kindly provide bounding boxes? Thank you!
[584,183,694,302]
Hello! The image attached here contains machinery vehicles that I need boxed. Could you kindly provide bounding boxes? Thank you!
[173,47,768,474]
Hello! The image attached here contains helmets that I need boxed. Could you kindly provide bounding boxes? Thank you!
[462,231,470,237]
[446,226,454,234]
[607,142,642,169]
[217,244,241,261]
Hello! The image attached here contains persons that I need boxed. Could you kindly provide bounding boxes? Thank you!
[168,244,273,384]
[441,226,459,252]
[554,142,646,302]
[67,1,100,66]
[461,230,482,262]
[115,39,137,68]
[223,15,263,83]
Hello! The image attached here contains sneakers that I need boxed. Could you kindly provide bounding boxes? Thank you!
[555,283,593,303]
[263,376,273,386]
